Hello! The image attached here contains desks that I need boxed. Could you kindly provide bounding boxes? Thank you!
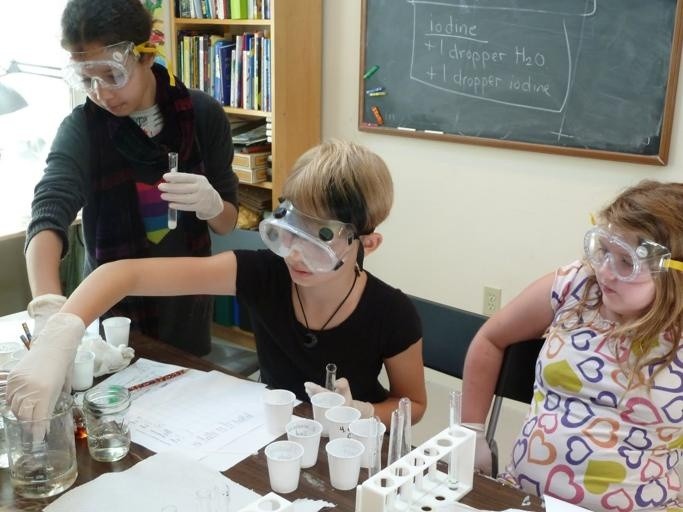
[1,313,544,512]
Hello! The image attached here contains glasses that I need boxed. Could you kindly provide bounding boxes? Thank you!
[64,40,136,95]
[259,199,364,275]
[584,213,683,284]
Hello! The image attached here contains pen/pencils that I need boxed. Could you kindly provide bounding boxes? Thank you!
[127,369,190,391]
[24,323,31,343]
[21,335,30,352]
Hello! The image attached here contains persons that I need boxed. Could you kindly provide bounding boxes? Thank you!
[458,180,682,512]
[24,1,238,366]
[4,144,427,450]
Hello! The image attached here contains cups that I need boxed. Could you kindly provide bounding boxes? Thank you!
[310,391,345,438]
[348,419,387,469]
[262,389,296,435]
[325,406,361,442]
[265,440,304,495]
[82,385,132,464]
[285,418,323,469]
[102,317,131,347]
[72,349,95,391]
[326,437,365,491]
[0,391,79,500]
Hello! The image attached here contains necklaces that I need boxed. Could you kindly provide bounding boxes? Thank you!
[295,273,359,348]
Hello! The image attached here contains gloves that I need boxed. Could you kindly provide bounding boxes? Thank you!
[461,423,492,478]
[157,172,224,219]
[6,312,85,445]
[27,294,72,396]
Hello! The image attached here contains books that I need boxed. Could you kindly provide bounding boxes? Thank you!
[175,0,271,21]
[173,27,271,112]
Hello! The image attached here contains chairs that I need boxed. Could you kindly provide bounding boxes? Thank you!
[411,294,489,461]
[490,339,540,473]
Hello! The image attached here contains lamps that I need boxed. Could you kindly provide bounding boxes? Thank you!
[0,60,77,119]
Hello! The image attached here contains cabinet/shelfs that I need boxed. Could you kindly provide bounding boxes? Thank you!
[168,0,323,228]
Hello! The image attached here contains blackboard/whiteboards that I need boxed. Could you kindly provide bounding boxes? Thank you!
[358,0,683,166]
